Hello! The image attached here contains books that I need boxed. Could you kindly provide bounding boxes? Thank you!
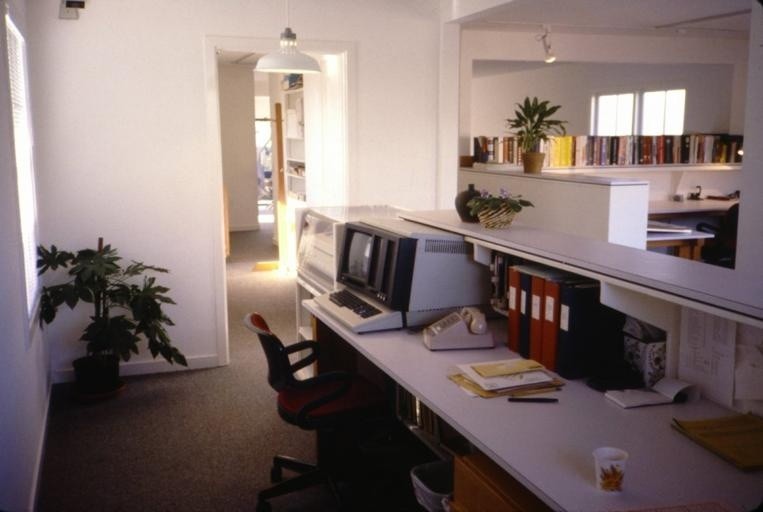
[398,386,464,457]
[604,375,702,410]
[473,131,744,168]
[488,253,522,309]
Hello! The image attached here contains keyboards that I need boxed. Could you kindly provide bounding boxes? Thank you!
[327,288,382,318]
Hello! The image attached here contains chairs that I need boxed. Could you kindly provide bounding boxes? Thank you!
[245,311,392,512]
[697,203,738,269]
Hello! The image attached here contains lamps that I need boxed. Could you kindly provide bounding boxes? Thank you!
[253,0,323,72]
[535,24,555,64]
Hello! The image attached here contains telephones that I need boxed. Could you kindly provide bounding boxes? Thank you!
[422,306,495,350]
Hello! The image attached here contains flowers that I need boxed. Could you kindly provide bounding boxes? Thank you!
[465,186,537,217]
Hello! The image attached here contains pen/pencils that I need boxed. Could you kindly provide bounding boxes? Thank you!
[508,397,558,403]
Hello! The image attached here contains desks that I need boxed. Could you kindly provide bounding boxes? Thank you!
[459,157,742,262]
[301,209,763,511]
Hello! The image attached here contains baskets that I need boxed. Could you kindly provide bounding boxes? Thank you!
[475,203,518,229]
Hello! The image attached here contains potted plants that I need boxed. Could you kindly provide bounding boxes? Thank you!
[36,233,188,399]
[505,94,569,175]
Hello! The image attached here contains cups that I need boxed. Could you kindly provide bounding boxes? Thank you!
[593,447,629,496]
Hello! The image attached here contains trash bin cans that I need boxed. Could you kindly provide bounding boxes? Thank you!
[410,461,453,512]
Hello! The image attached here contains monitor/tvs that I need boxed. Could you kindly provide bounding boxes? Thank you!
[344,230,374,285]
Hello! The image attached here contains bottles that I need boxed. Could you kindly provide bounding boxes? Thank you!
[455,185,481,224]
